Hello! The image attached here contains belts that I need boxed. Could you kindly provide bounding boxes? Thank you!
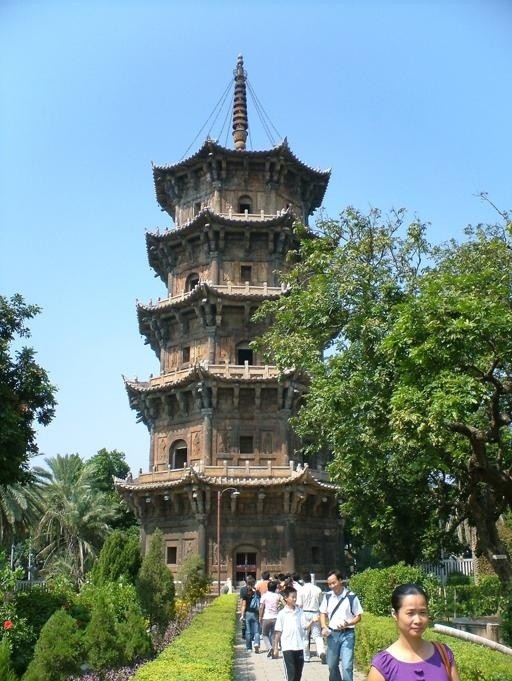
[329,628,355,632]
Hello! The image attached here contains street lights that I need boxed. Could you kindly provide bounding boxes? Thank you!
[216,485,240,595]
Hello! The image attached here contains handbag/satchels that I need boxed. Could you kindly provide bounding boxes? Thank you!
[323,636,329,647]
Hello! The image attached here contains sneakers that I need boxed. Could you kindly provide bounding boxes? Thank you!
[253,644,260,653]
[246,649,252,654]
[319,653,327,664]
[267,647,273,658]
[303,656,310,662]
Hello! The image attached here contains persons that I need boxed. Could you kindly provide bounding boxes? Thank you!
[367,583,461,681]
[239,569,363,681]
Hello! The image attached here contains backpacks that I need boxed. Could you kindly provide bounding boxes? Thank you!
[249,590,260,609]
